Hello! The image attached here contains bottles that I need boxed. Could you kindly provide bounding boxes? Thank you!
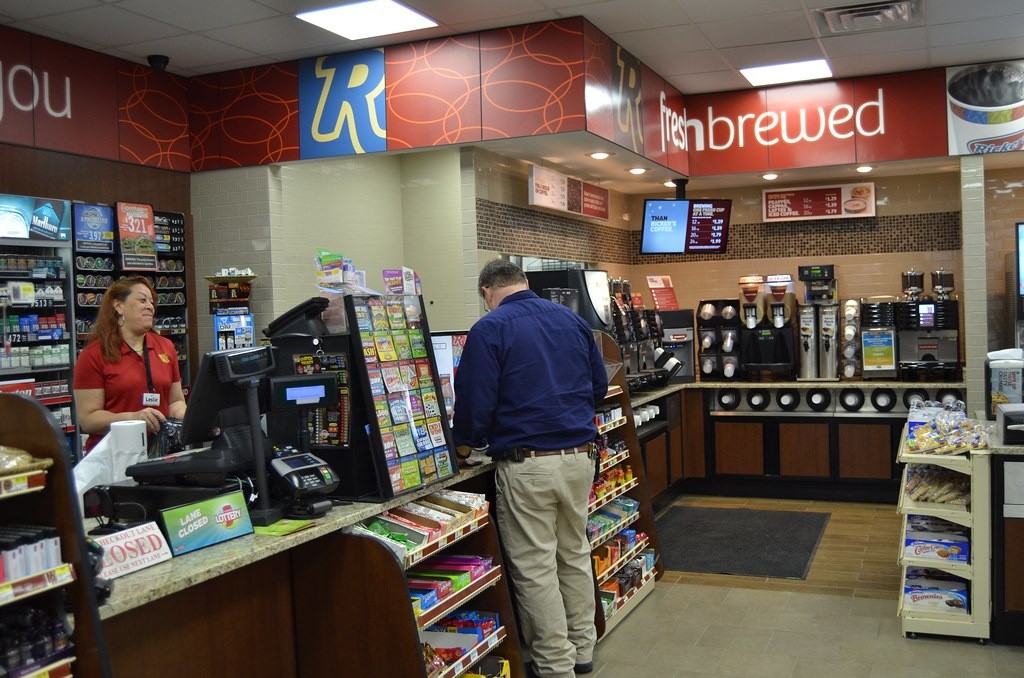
[440,374,453,421]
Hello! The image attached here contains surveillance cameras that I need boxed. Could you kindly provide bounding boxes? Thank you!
[147,55,169,73]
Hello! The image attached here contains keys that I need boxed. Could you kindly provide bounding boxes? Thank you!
[588,442,597,460]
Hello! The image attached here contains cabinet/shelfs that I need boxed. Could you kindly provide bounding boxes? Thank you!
[291,488,525,678]
[0,269,74,438]
[0,391,110,678]
[895,404,991,646]
[74,252,191,398]
[575,328,664,647]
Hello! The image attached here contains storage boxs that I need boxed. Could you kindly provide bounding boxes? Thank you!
[586,497,654,620]
[341,490,509,678]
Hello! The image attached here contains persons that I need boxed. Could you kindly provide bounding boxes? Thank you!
[451,259,608,678]
[72,276,187,457]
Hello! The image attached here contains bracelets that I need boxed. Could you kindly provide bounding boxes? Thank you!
[455,449,471,460]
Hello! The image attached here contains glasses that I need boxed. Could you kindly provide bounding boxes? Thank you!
[483,285,491,312]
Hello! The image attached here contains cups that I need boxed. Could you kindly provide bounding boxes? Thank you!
[720,394,956,406]
[947,63,1024,156]
[722,306,736,377]
[843,198,868,213]
[844,300,858,378]
[699,303,716,374]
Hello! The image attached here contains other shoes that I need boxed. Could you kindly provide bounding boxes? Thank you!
[523,662,540,678]
[573,661,593,674]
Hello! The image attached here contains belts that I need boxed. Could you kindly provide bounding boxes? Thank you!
[523,443,592,457]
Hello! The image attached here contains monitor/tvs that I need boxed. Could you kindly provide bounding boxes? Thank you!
[180,346,280,445]
[1014,222,1024,297]
[640,199,732,255]
